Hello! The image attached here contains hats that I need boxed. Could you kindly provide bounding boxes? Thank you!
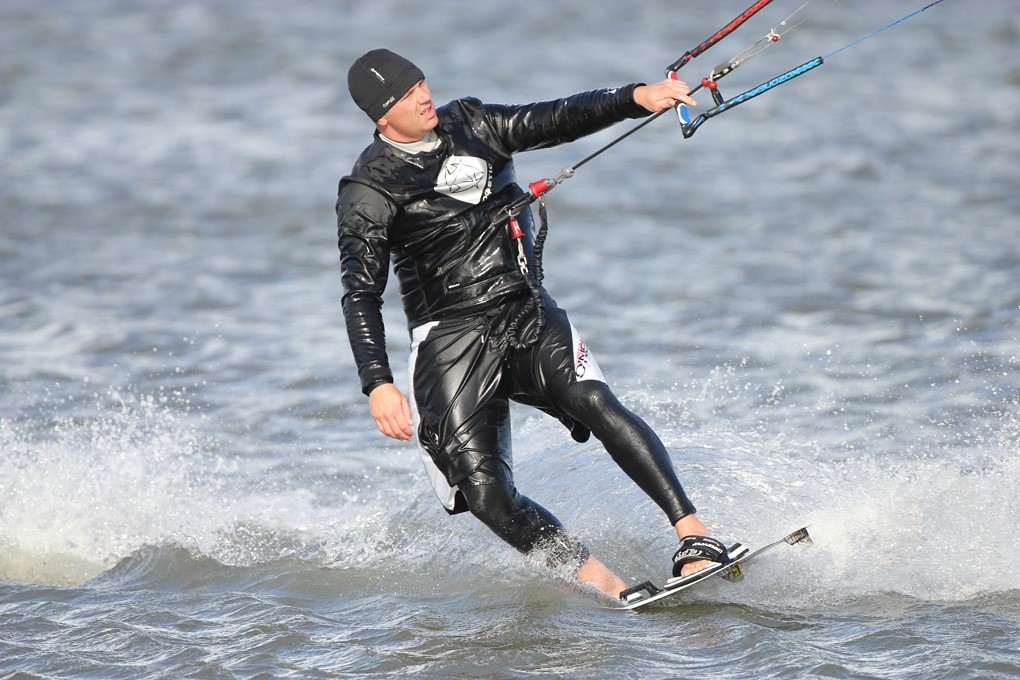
[347,48,425,122]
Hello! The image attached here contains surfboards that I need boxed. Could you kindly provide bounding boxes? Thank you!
[596,519,815,614]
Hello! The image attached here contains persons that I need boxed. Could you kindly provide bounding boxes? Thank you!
[334,47,726,602]
